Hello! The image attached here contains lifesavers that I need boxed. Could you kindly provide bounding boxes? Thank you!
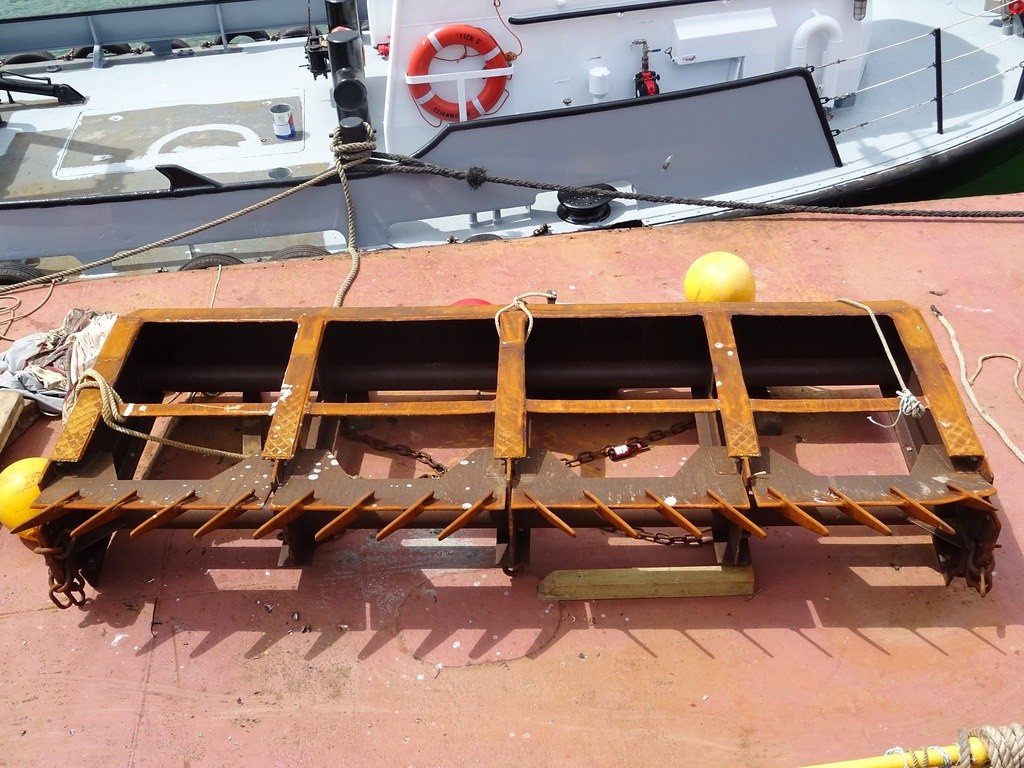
[406,24,508,123]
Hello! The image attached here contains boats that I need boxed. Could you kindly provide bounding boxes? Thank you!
[0,0,1024,288]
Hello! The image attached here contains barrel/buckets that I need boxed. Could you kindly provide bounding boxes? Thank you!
[270,104,296,139]
